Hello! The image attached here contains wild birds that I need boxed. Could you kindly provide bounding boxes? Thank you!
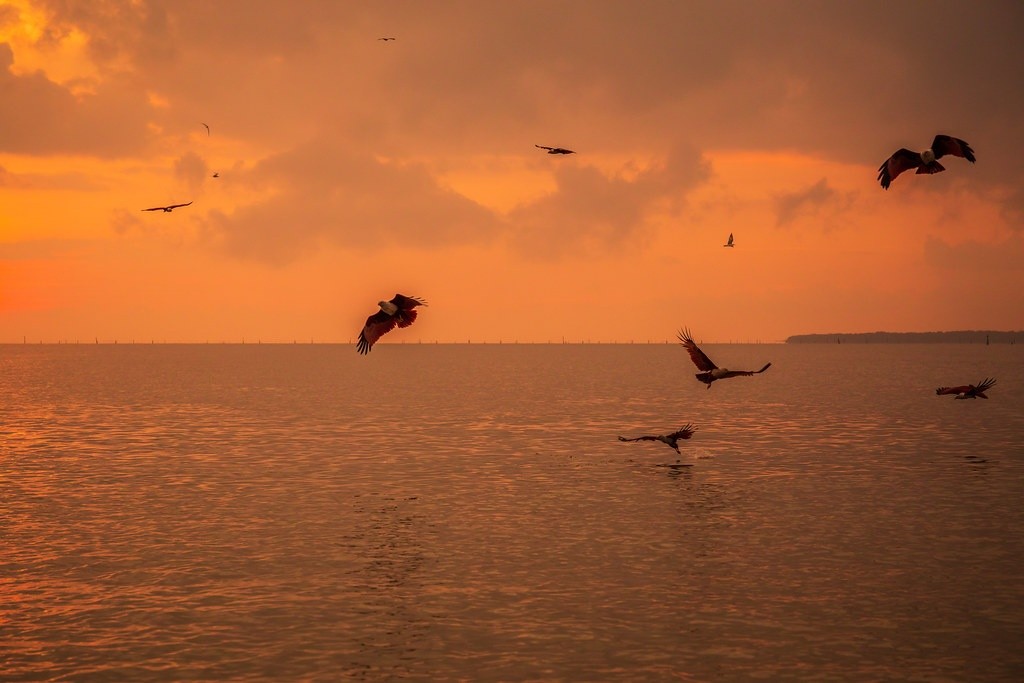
[723,232,735,248]
[355,293,429,355]
[535,144,576,155]
[378,37,396,41]
[213,173,219,177]
[936,376,998,400]
[877,134,977,190]
[676,325,772,390]
[202,122,210,136]
[141,201,194,212]
[616,421,699,454]
